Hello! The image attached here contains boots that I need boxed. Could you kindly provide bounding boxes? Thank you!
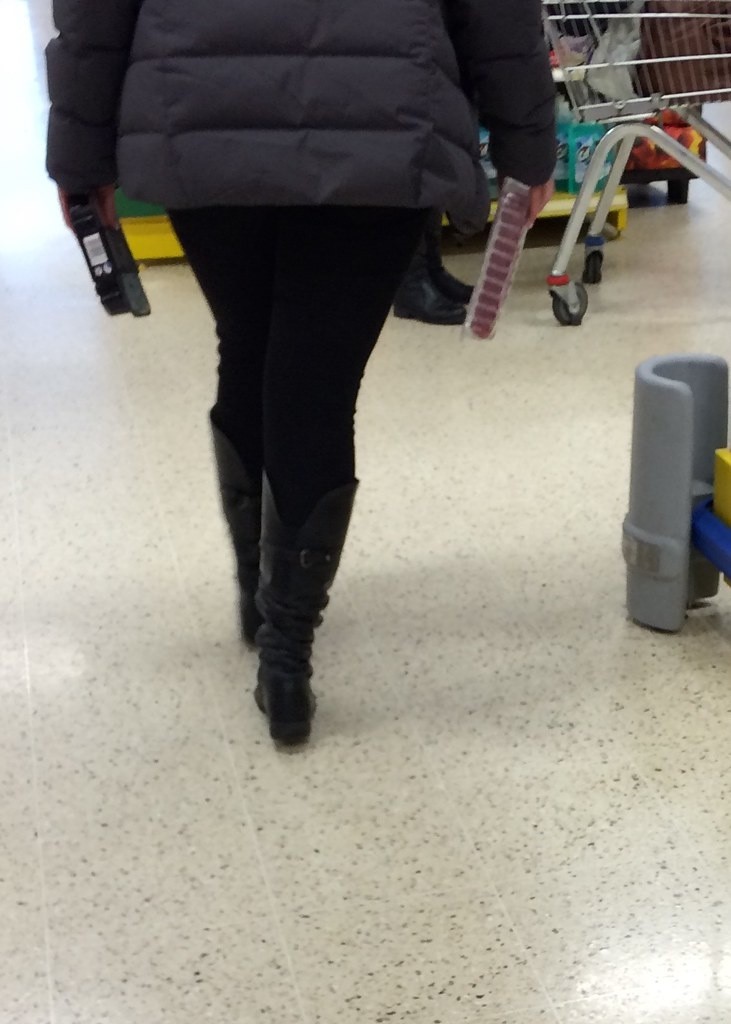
[209,403,361,744]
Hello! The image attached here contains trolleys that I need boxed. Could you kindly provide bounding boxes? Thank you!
[541,0,731,327]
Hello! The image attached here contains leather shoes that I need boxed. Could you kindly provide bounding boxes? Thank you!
[396,258,473,324]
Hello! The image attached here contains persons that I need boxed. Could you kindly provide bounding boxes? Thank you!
[47,0,557,749]
